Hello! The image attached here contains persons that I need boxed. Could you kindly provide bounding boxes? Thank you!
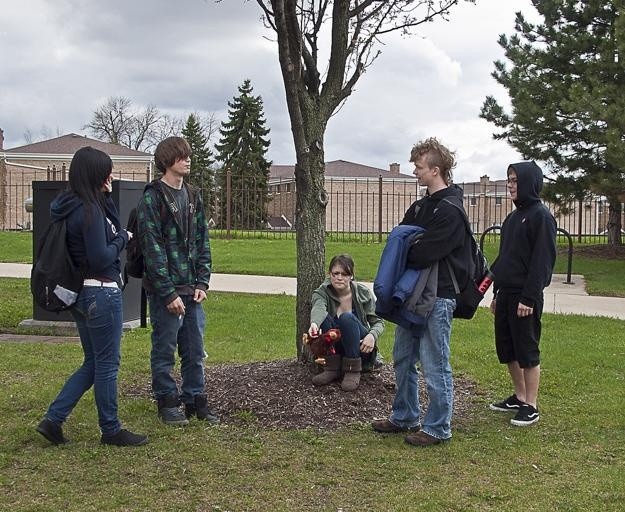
[371,138,472,447]
[309,255,385,391]
[490,160,557,427]
[136,137,221,426]
[33,146,146,447]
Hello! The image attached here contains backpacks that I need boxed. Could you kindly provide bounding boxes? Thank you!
[124,192,166,278]
[32,211,88,312]
[454,196,488,319]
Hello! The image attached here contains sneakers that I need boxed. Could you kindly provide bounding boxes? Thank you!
[35,418,69,445]
[489,394,522,412]
[101,429,148,447]
[511,403,540,425]
[371,418,421,432]
[404,428,439,445]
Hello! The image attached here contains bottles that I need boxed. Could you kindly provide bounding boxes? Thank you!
[477,269,497,294]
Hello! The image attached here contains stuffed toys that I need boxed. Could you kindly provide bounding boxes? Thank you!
[302,328,341,365]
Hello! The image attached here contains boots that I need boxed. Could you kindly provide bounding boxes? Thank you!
[312,354,343,386]
[184,393,219,423]
[157,392,189,425]
[342,357,362,392]
